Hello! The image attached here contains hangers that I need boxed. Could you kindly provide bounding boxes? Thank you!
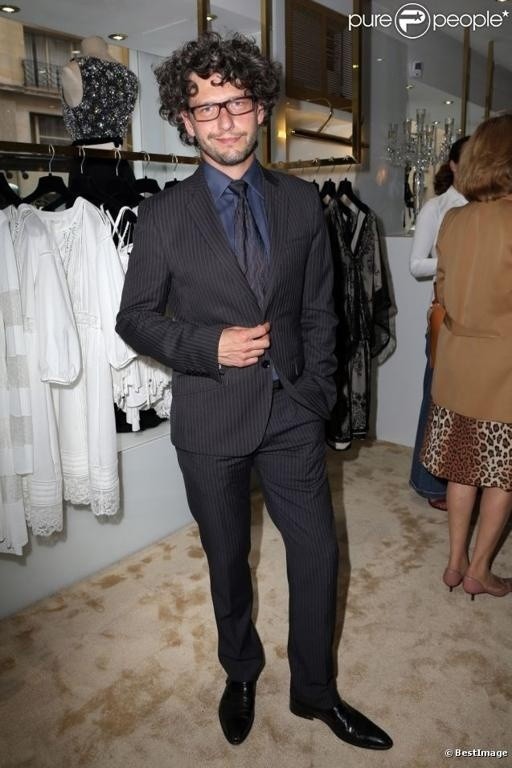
[267,154,371,218]
[0,140,200,237]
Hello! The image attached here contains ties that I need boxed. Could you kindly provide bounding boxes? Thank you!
[228,177,272,313]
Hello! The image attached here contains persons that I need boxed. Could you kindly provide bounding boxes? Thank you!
[408,135,471,511]
[419,115,512,602]
[115,30,394,751]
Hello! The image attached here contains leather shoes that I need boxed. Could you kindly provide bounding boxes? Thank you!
[218,663,259,746]
[421,494,449,513]
[287,679,395,751]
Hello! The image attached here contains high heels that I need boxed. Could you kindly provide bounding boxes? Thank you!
[464,568,512,603]
[442,563,465,594]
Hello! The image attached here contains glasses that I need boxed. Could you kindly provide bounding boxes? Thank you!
[181,93,261,123]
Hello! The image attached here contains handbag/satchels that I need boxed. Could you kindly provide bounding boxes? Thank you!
[425,305,447,370]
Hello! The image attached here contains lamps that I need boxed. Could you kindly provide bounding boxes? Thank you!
[381,108,455,237]
